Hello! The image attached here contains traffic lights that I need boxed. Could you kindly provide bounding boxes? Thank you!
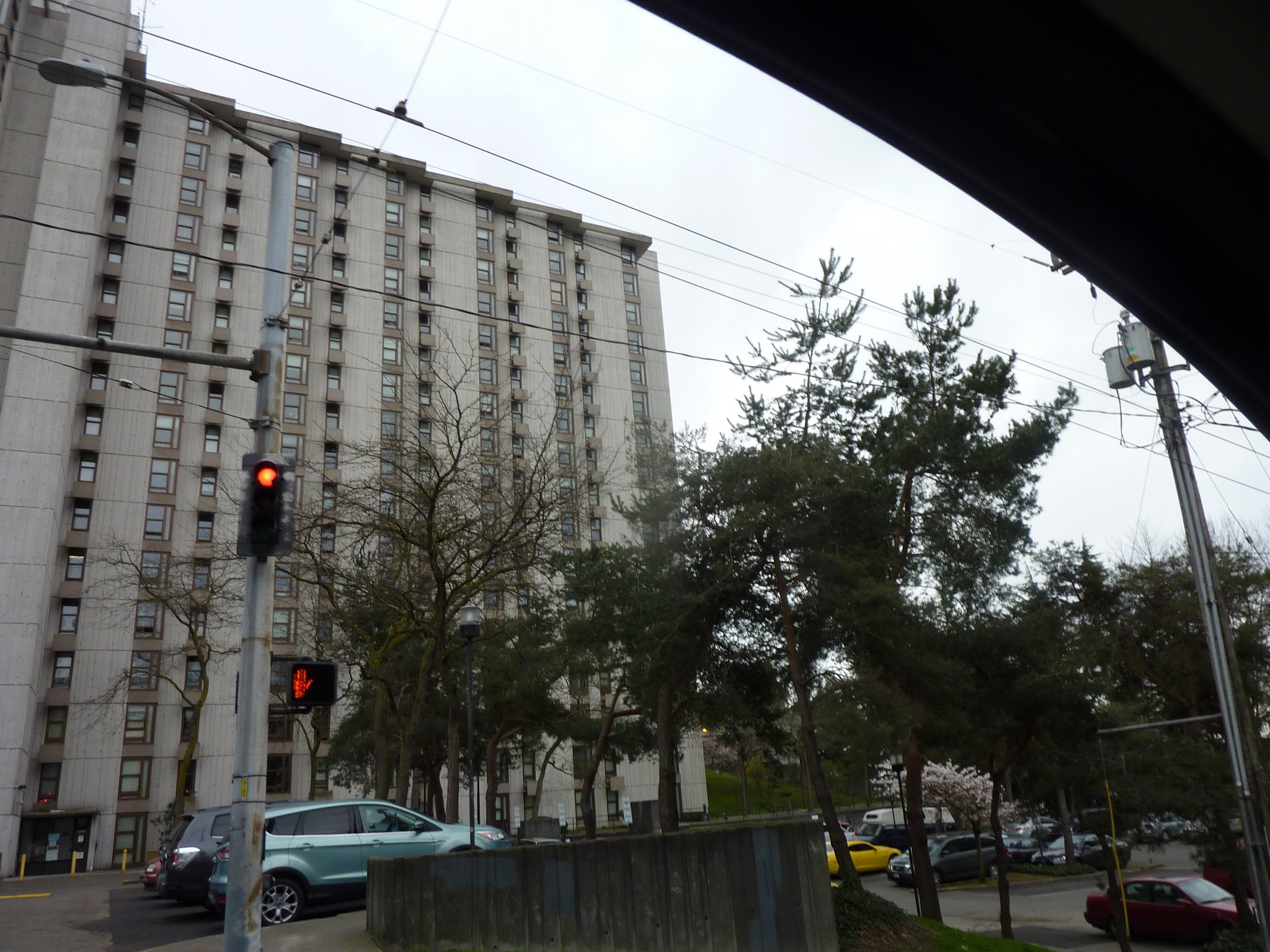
[236,452,295,556]
[285,661,338,706]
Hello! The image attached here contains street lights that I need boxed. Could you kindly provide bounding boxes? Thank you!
[459,606,487,952]
[0,57,296,952]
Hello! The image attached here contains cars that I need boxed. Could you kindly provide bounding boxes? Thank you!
[208,799,512,925]
[514,837,562,846]
[823,822,855,842]
[824,841,902,879]
[140,859,162,887]
[1005,815,1073,838]
[1083,877,1261,949]
[155,799,312,916]
[887,835,1010,887]
[1032,833,1131,871]
[1073,807,1216,845]
[1204,829,1260,896]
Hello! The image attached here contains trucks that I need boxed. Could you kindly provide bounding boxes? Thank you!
[853,806,959,854]
